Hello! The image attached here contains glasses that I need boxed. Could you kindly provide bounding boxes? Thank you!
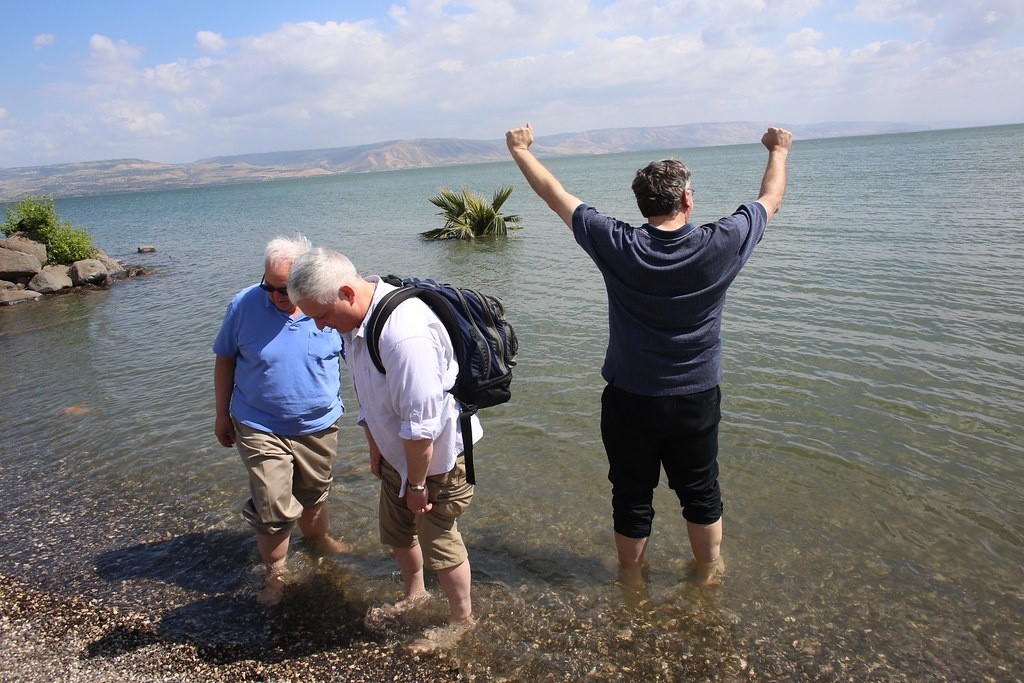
[259,274,288,297]
[687,188,695,196]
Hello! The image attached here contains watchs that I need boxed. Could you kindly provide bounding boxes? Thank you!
[407,479,426,492]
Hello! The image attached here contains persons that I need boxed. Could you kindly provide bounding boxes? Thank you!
[213,232,354,610]
[506,125,794,588]
[284,247,520,658]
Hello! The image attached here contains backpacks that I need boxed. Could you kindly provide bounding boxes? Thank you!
[364,274,518,416]
